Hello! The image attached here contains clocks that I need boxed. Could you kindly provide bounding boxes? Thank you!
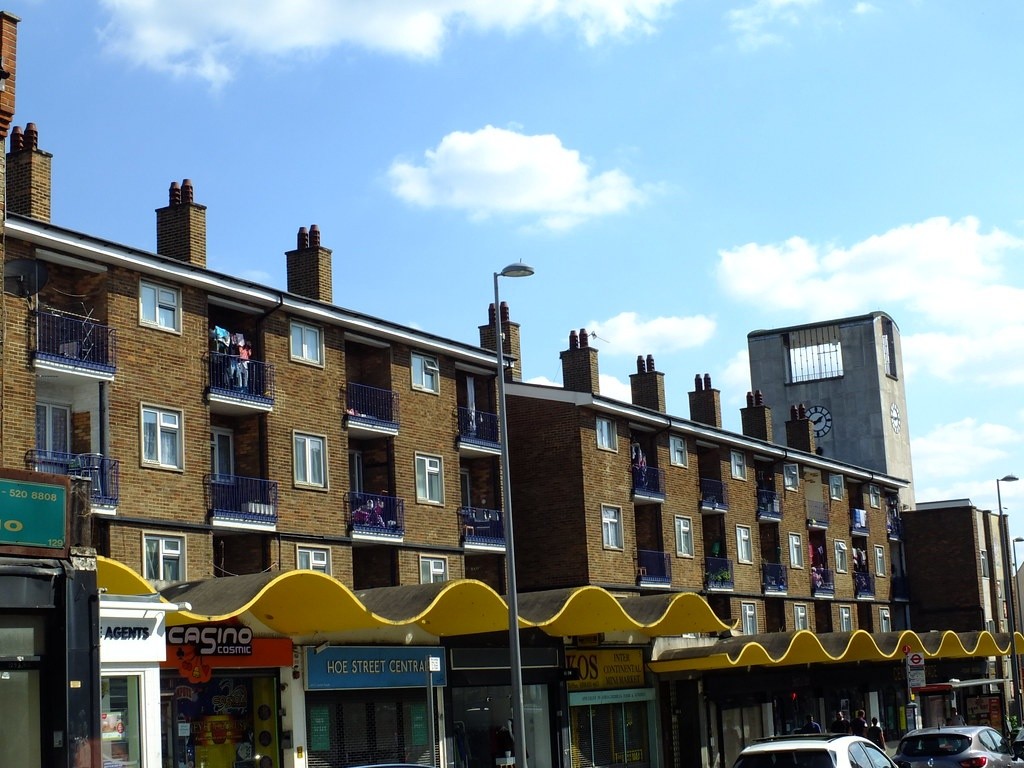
[805,406,832,438]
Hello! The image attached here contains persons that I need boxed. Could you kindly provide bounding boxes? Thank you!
[830,711,851,735]
[801,713,823,733]
[946,707,967,727]
[866,717,886,752]
[850,710,869,739]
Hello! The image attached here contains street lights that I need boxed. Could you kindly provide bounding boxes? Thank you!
[492,264,534,768]
[997,474,1024,729]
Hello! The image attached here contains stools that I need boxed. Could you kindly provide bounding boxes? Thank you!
[69,452,104,498]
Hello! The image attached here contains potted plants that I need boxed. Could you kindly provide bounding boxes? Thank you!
[241,498,277,516]
[708,568,733,587]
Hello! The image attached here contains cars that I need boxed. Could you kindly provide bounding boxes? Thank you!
[733,733,902,768]
[892,725,1024,768]
